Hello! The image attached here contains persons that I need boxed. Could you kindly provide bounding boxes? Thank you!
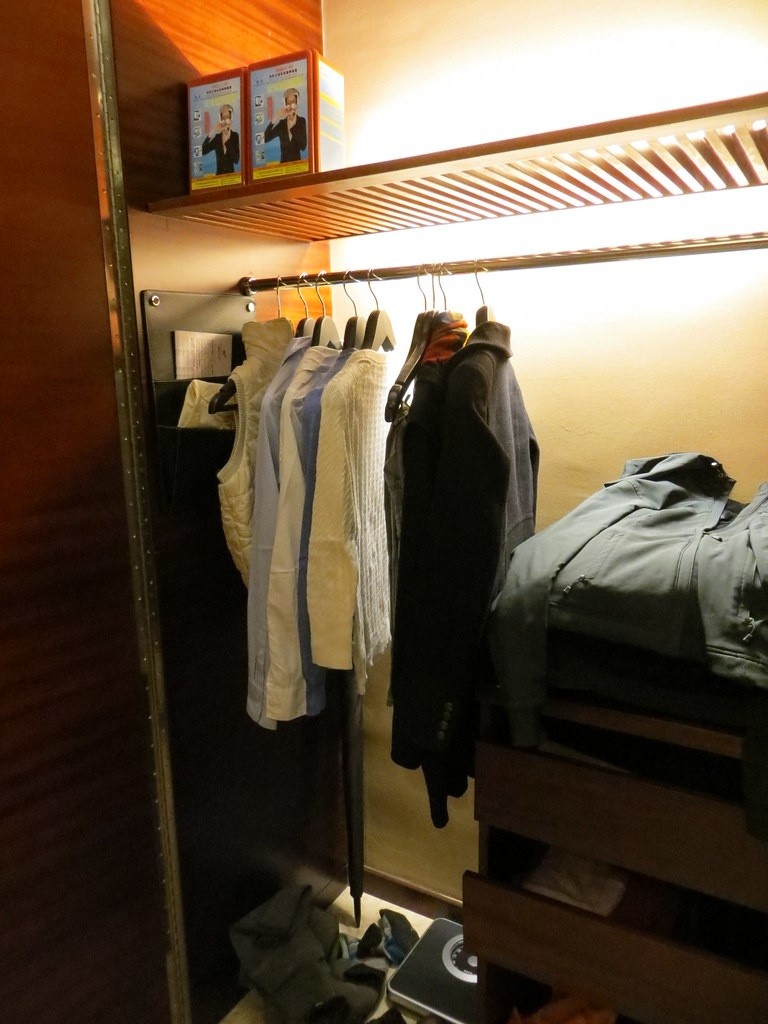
[265,94,307,164]
[202,111,239,175]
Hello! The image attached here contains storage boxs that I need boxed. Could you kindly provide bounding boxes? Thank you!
[246,48,345,185]
[187,67,246,195]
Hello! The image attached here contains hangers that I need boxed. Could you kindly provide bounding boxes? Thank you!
[209,274,496,427]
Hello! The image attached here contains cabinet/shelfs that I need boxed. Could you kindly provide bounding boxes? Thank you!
[0,0,767,1024]
